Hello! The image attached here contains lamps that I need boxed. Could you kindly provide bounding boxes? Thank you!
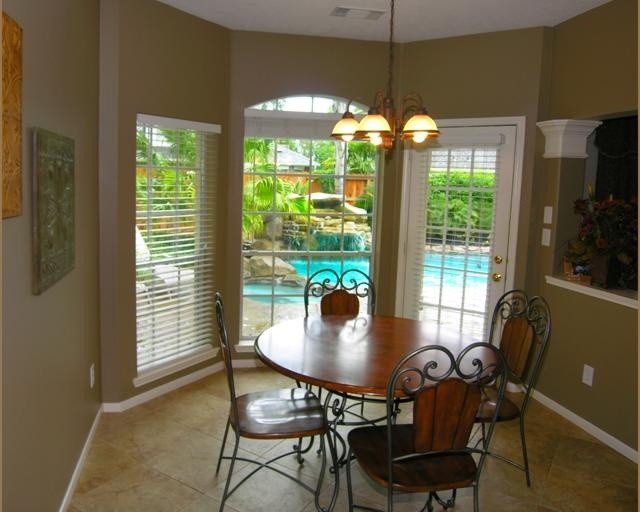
[329,0,440,146]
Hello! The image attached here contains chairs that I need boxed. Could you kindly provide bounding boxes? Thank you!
[293,269,376,465]
[346,342,509,512]
[215,288,339,512]
[135,225,194,304]
[476,290,552,488]
[135,281,152,304]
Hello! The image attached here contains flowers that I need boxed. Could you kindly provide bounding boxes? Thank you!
[573,184,637,265]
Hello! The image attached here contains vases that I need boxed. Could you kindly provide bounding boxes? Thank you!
[591,256,609,288]
